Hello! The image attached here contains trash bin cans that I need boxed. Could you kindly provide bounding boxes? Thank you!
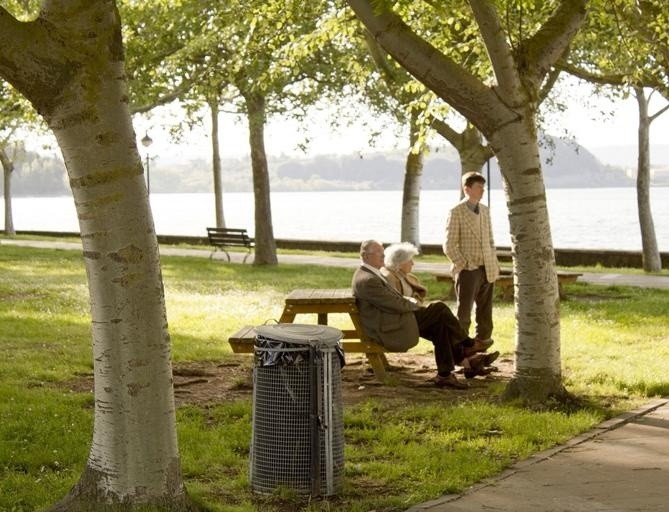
[251,324,346,497]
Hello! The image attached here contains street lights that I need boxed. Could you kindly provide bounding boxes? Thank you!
[142,128,153,195]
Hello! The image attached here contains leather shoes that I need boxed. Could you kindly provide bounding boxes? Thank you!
[465,350,501,378]
[436,373,469,390]
[462,337,495,354]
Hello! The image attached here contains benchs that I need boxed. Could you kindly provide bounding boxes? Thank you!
[206,226,255,263]
[227,326,407,381]
[436,269,584,300]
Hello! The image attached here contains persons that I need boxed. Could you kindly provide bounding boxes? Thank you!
[377,243,500,379]
[442,172,502,376]
[351,240,493,390]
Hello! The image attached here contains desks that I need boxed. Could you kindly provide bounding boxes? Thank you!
[278,288,389,381]
